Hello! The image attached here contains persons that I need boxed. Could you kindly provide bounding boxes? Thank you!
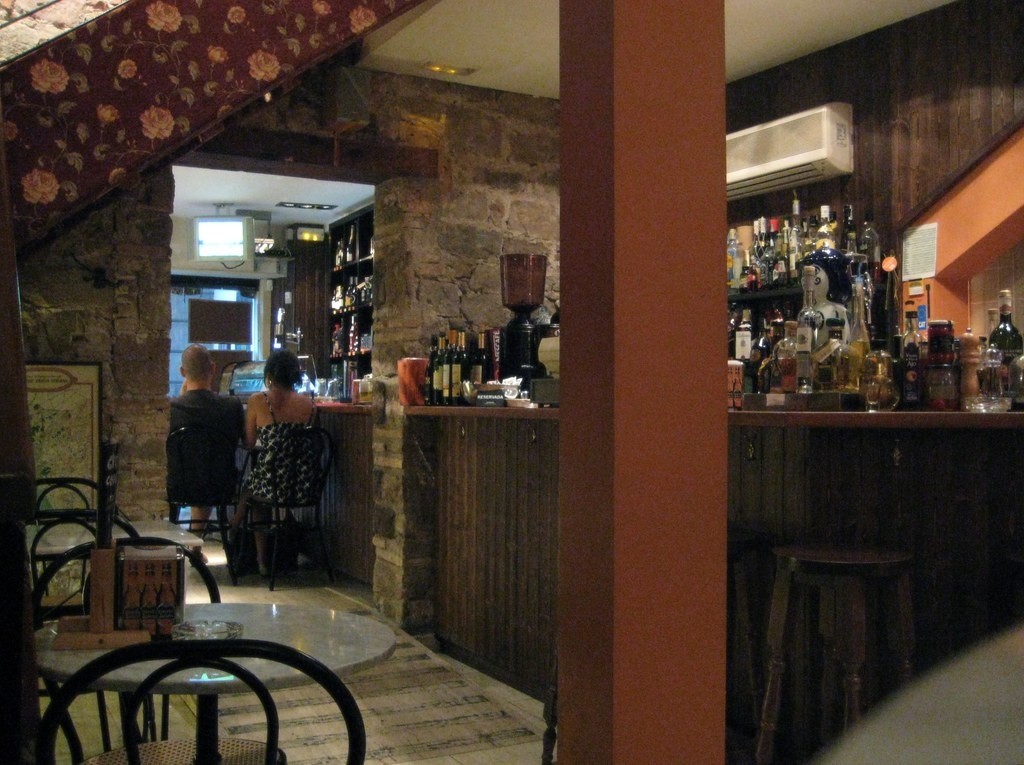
[229,351,322,578]
[166,344,245,565]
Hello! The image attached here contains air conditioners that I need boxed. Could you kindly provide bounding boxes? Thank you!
[726,102,855,203]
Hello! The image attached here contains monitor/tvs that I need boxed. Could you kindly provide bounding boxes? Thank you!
[192,215,248,261]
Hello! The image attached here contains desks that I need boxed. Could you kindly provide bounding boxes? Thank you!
[26,519,203,753]
[34,603,396,765]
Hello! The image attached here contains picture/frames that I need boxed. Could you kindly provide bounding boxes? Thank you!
[26,359,102,515]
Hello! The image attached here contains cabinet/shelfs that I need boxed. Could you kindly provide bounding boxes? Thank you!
[326,194,375,403]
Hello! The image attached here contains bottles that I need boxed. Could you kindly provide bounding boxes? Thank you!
[327,224,375,360]
[423,327,493,408]
[890,312,926,408]
[124,563,179,638]
[734,265,894,411]
[726,195,882,294]
[989,290,1024,379]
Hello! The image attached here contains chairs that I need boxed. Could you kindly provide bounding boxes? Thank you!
[167,426,237,587]
[34,639,367,765]
[30,477,98,520]
[238,428,334,594]
[31,537,222,754]
[25,507,153,765]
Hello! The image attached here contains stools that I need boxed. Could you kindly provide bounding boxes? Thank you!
[750,544,917,765]
